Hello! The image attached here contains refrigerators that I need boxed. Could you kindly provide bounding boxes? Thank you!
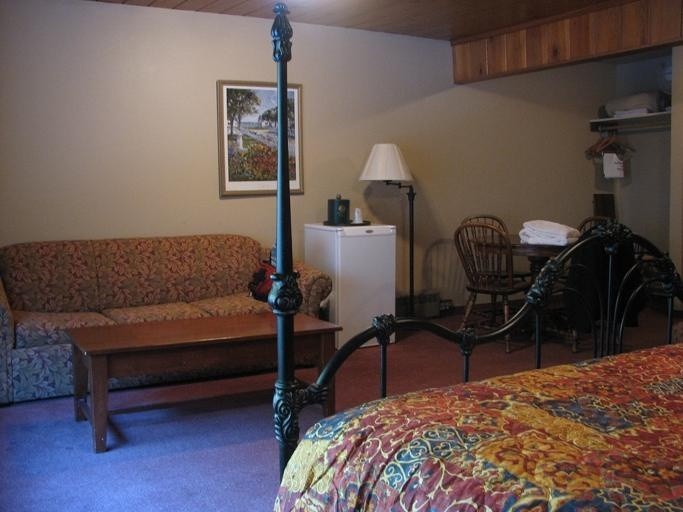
[301,221,397,352]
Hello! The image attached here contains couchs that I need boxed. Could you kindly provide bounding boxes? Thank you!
[0,234,333,405]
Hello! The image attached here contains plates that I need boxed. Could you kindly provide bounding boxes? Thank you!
[323,219,370,226]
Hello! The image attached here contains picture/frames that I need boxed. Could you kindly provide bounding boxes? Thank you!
[216,79,305,198]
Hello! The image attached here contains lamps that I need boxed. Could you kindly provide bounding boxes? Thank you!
[359,143,416,319]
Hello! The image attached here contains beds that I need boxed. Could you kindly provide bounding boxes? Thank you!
[267,1,683,512]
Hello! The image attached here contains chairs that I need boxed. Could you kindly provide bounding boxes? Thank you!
[454,223,532,353]
[459,214,532,323]
[577,216,613,234]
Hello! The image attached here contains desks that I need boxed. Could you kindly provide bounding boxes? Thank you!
[469,234,570,346]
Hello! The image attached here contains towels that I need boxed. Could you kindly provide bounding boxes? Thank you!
[519,219,581,246]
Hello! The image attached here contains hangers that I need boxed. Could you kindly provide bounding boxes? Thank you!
[584,125,637,161]
